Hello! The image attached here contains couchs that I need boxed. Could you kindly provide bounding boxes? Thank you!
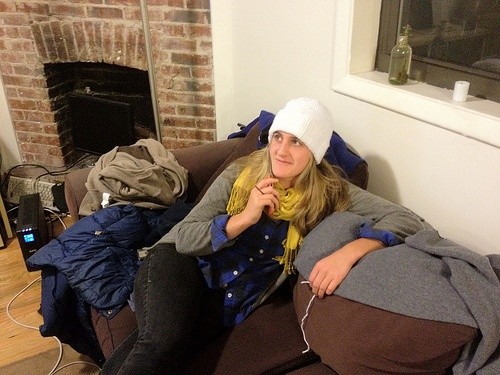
[63,135,500,375]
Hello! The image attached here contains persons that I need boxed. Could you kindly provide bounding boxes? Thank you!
[96,98,424,375]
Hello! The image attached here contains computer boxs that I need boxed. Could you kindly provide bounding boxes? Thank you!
[16,192,51,272]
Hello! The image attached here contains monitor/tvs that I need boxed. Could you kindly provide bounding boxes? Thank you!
[67,91,136,156]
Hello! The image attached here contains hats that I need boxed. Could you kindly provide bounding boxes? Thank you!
[267,95,332,166]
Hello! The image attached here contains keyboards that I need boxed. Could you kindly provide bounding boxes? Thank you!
[6,176,61,212]
